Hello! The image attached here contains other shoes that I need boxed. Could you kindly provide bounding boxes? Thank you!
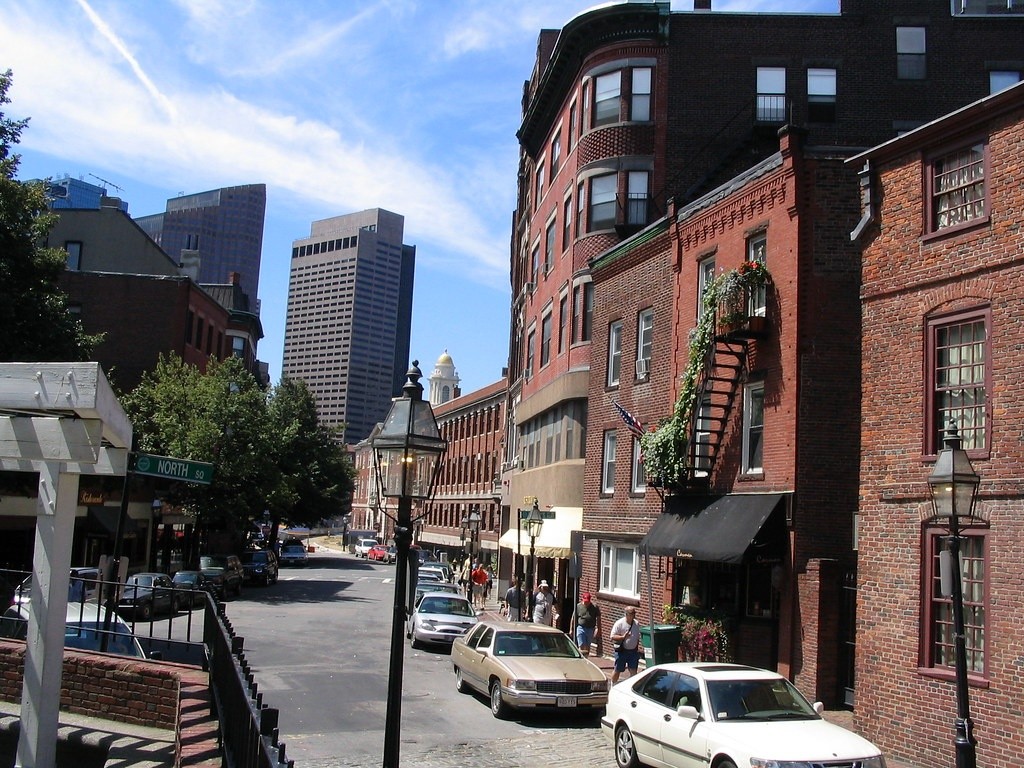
[480,607,484,610]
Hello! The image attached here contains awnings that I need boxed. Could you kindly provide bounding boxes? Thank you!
[641,491,787,570]
[499,504,583,560]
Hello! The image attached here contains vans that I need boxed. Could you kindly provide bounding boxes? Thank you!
[14,567,99,605]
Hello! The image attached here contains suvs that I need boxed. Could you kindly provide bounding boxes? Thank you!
[0,602,162,660]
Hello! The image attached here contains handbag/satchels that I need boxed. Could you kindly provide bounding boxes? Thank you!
[613,643,622,652]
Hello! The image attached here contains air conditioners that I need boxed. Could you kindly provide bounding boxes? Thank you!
[636,359,651,374]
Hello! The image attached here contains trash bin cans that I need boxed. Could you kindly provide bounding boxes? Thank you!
[640,624,685,668]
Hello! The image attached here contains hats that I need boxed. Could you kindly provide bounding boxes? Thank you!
[583,592,591,602]
[537,579,549,588]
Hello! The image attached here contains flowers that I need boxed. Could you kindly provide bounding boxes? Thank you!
[738,259,774,288]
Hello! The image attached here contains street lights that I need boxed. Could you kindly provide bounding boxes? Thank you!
[926,418,982,768]
[461,509,481,603]
[371,360,448,768]
[342,517,348,551]
[525,499,544,621]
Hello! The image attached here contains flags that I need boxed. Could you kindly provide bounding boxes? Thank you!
[615,402,647,462]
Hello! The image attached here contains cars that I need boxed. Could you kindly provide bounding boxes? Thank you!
[279,538,309,567]
[450,621,609,719]
[195,555,244,599]
[172,571,216,606]
[353,538,483,650]
[601,662,886,768]
[114,572,186,620]
[241,549,279,586]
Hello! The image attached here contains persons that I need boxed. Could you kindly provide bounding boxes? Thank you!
[458,554,496,602]
[68,570,88,603]
[472,563,487,610]
[274,538,283,556]
[504,579,527,622]
[569,593,602,657]
[609,606,640,685]
[525,580,559,626]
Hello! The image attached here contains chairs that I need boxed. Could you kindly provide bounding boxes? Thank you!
[526,638,546,654]
[423,603,435,612]
[741,685,775,712]
[715,691,750,720]
[496,636,519,655]
